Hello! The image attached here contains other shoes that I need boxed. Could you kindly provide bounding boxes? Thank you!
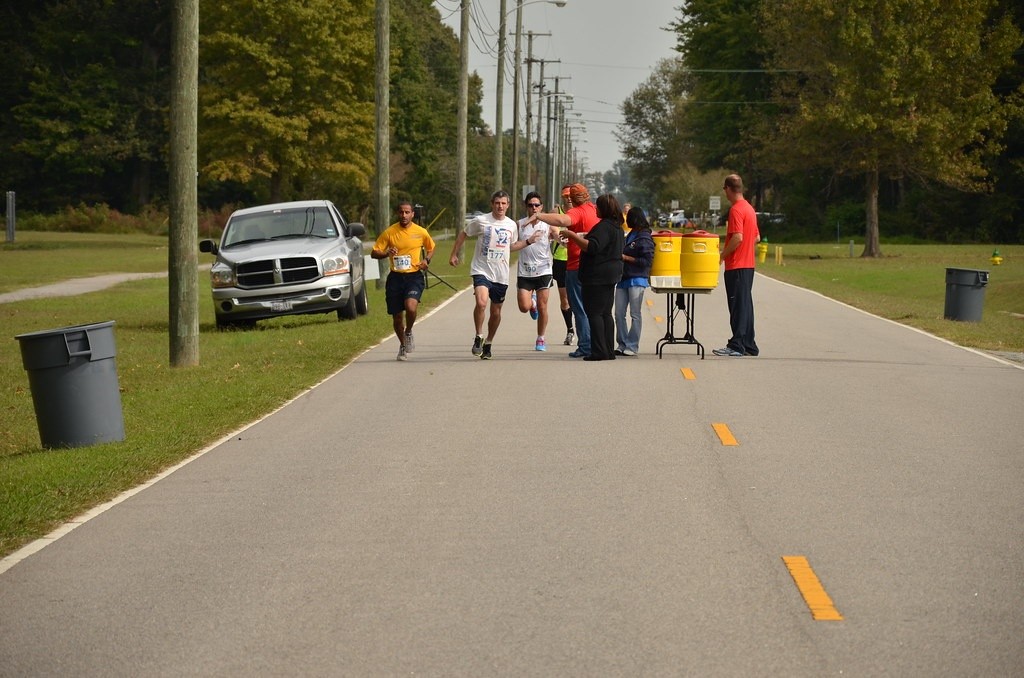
[583,354,600,361]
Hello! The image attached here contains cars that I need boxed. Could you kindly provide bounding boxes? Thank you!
[656,208,720,230]
[198,198,369,328]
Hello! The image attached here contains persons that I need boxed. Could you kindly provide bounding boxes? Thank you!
[559,194,625,361]
[613,206,656,356]
[370,202,435,362]
[548,185,576,345]
[523,183,602,358]
[449,191,542,360]
[714,172,759,358]
[516,192,568,350]
[621,203,632,233]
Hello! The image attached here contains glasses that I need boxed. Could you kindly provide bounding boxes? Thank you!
[527,203,542,207]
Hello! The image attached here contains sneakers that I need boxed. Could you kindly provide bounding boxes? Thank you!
[536,337,545,351]
[614,349,623,355]
[623,349,637,355]
[405,328,414,353]
[472,336,484,357]
[729,351,743,356]
[569,348,590,357]
[481,342,492,360]
[530,294,538,320]
[564,333,576,345]
[397,344,407,361]
[713,348,735,356]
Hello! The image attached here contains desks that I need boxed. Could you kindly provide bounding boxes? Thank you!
[649,287,713,360]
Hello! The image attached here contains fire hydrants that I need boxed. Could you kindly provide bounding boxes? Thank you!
[988,250,1003,266]
[756,236,770,264]
[654,221,657,228]
[668,218,672,229]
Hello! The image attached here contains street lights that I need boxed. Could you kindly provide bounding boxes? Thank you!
[525,93,591,217]
[492,0,567,201]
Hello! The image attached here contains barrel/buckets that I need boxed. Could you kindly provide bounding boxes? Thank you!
[679,229,720,289]
[650,229,683,288]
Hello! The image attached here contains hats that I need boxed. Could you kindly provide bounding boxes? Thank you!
[562,187,570,196]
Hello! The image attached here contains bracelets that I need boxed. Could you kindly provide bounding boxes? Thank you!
[526,239,531,246]
[426,257,430,264]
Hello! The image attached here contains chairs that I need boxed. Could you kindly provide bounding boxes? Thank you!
[242,225,265,240]
[311,218,331,236]
